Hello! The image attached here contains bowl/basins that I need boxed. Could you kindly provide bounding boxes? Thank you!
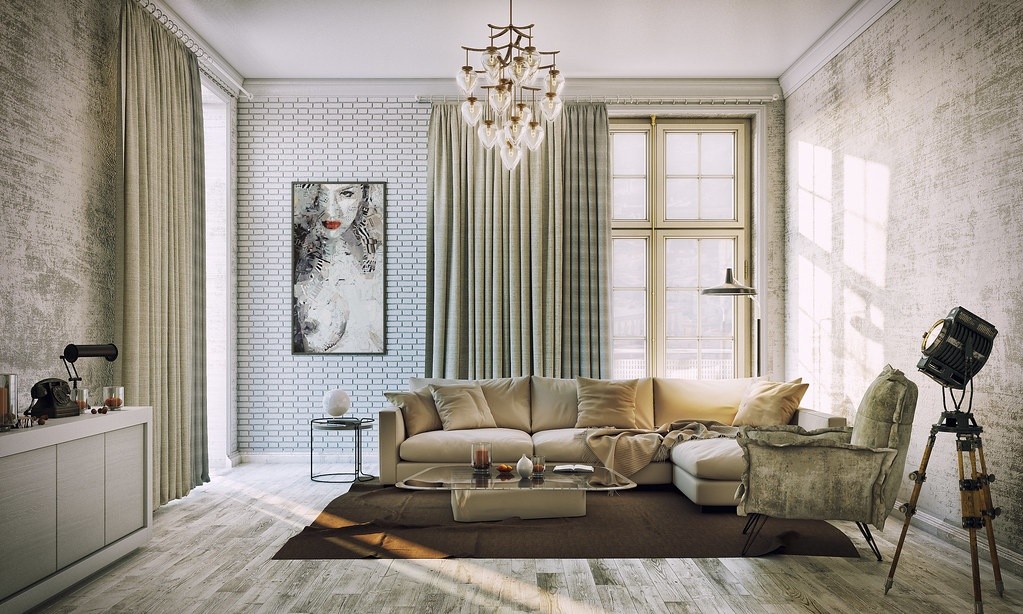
[496,467,513,473]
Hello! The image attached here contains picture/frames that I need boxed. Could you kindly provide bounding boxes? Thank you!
[292,181,387,355]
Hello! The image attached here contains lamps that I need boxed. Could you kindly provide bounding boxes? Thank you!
[454,0,565,169]
[702,269,762,377]
[60,343,118,396]
[916,307,999,395]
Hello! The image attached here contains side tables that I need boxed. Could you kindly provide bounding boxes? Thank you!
[309,417,375,483]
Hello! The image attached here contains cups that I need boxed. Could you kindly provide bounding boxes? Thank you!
[103,386,124,411]
[70,388,88,414]
[470,442,494,474]
[0,373,18,428]
[530,455,545,478]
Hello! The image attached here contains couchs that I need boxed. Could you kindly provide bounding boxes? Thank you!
[380,375,848,507]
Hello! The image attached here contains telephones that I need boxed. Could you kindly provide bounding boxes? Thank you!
[31,378,80,418]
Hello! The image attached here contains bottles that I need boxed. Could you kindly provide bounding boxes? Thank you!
[517,454,533,479]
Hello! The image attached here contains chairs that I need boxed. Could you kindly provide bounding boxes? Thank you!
[735,366,917,561]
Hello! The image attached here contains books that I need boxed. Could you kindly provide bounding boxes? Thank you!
[553,464,594,473]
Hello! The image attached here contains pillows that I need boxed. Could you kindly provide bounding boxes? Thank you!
[384,387,441,435]
[427,381,497,430]
[729,380,810,426]
[738,377,803,409]
[575,375,639,428]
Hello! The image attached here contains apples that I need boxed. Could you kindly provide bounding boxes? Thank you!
[105,398,123,407]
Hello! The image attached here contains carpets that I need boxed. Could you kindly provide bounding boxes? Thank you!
[273,477,860,560]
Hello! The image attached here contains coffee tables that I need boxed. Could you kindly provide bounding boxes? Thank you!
[396,464,636,521]
